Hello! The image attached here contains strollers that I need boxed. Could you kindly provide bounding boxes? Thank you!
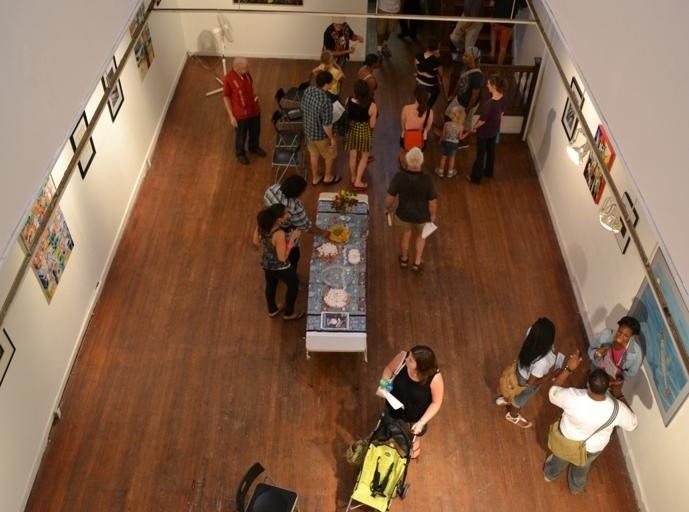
[337,414,417,512]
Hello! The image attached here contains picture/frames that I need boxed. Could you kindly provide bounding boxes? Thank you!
[625,242,689,427]
[69,110,96,180]
[583,123,617,205]
[18,173,75,305]
[561,77,585,142]
[101,55,124,123]
[614,192,639,254]
[0,326,16,387]
[320,311,350,330]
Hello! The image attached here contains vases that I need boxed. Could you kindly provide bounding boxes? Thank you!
[340,206,347,216]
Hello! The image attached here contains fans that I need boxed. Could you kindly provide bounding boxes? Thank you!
[206,11,235,95]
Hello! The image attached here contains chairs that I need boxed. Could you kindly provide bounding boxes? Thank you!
[235,462,299,512]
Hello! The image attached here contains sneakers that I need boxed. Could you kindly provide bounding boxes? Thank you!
[446,168,459,179]
[236,151,250,165]
[248,145,266,157]
[377,44,392,62]
[433,167,445,178]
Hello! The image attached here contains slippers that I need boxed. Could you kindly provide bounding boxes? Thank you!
[283,311,306,321]
[408,437,422,460]
[312,173,324,186]
[322,175,342,185]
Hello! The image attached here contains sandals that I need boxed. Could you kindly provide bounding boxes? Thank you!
[505,411,533,430]
[399,254,409,269]
[411,259,425,273]
[496,396,511,406]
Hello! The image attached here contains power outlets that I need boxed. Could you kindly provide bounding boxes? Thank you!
[186,50,192,59]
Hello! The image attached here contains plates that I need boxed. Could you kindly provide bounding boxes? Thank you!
[316,215,361,308]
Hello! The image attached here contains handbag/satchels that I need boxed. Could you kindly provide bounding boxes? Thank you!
[546,414,588,467]
[498,358,531,404]
[337,97,355,137]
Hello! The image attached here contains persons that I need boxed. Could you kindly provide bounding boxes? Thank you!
[299,14,377,188]
[382,147,438,273]
[496,317,643,495]
[376,346,445,460]
[398,0,525,182]
[375,2,402,53]
[221,56,266,164]
[252,203,305,321]
[262,176,332,311]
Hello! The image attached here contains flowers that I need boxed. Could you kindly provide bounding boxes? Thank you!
[330,189,359,210]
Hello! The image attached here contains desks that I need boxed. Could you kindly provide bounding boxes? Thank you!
[306,193,369,363]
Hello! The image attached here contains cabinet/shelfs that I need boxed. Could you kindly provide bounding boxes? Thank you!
[271,91,309,184]
